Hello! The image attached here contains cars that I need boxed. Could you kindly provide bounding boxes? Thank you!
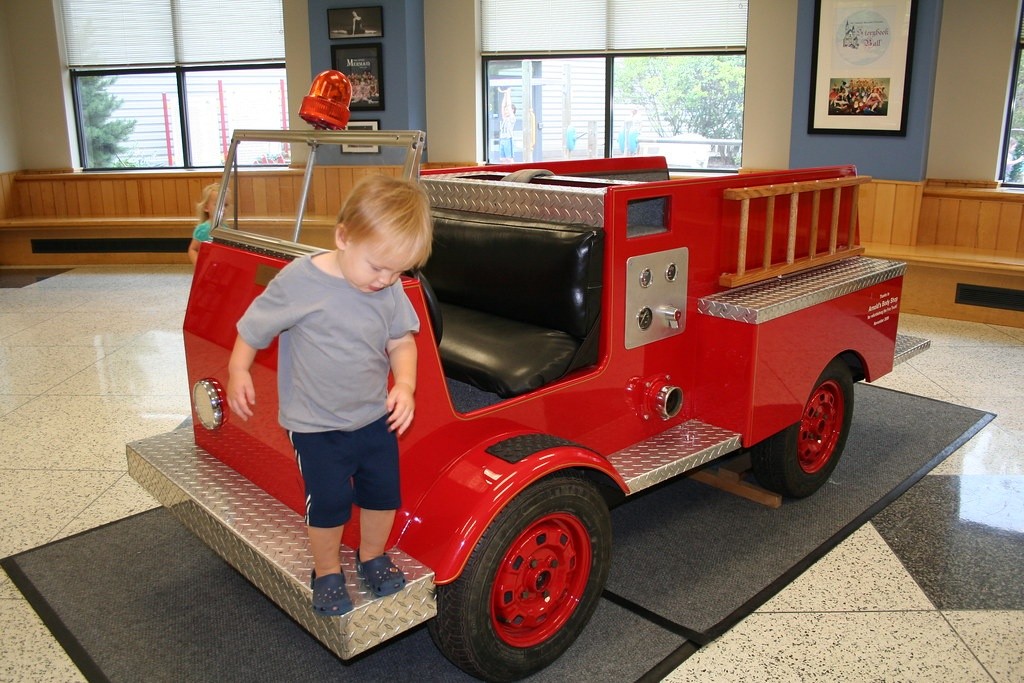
[121,68,933,683]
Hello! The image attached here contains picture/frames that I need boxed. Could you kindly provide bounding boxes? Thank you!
[327,5,383,40]
[340,119,382,154]
[808,0,919,137]
[330,43,386,112]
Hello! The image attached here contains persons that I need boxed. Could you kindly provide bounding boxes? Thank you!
[224,163,437,617]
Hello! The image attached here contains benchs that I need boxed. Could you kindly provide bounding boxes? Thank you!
[0,173,339,266]
[861,192,1024,330]
[416,205,605,398]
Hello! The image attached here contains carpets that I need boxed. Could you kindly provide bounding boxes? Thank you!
[0,378,998,683]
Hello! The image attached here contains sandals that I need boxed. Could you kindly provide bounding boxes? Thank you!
[310,565,352,616]
[355,549,406,597]
